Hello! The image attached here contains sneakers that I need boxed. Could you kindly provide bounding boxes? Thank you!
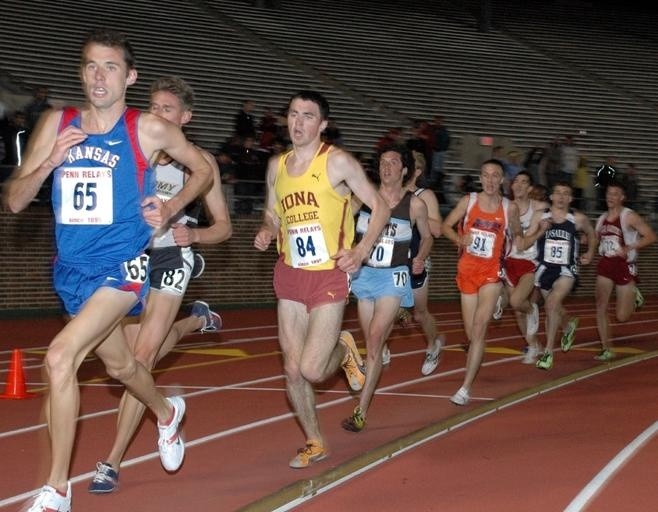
[27,396,186,512]
[191,300,223,334]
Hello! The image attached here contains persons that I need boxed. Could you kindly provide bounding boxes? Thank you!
[0,88,645,257]
[380,149,444,379]
[344,142,433,432]
[2,30,214,512]
[505,172,551,366]
[254,88,394,471]
[444,160,521,406]
[594,185,655,361]
[522,183,598,372]
[89,74,230,495]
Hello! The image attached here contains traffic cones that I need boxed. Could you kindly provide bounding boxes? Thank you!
[0,347,42,400]
